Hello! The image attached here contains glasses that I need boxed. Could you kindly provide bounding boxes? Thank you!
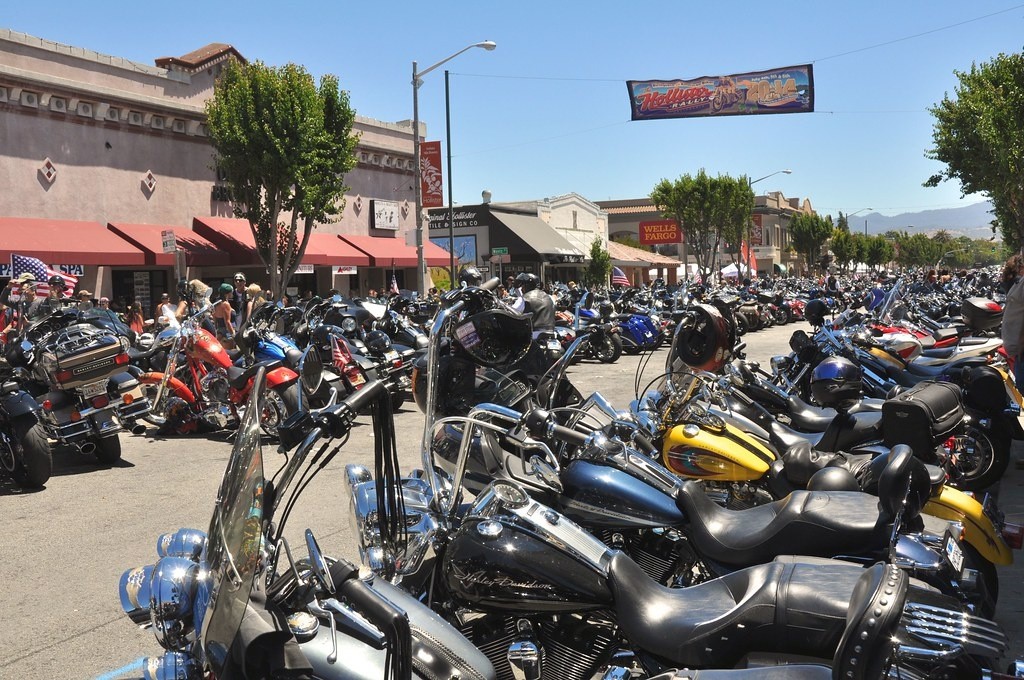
[161,297,170,300]
[235,279,245,282]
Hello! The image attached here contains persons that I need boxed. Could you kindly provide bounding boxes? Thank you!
[824,273,840,296]
[1002,244,1024,396]
[498,276,622,304]
[872,264,967,286]
[458,266,483,294]
[761,276,773,290]
[0,273,452,344]
[512,273,555,340]
[720,280,727,290]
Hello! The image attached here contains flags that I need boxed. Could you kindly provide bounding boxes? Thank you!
[8,253,77,306]
[391,274,399,295]
[613,267,630,287]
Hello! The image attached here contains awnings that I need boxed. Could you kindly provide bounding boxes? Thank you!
[0,217,456,269]
[488,208,585,262]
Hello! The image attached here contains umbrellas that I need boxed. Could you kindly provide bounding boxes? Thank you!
[720,262,757,278]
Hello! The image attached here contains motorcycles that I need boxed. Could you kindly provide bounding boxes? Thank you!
[0,253,1024,680]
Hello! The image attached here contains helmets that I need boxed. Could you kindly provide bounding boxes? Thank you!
[805,300,832,327]
[810,356,864,405]
[364,331,392,355]
[458,267,482,287]
[805,467,860,491]
[313,324,344,364]
[140,333,154,348]
[514,273,537,295]
[449,310,535,371]
[677,300,738,370]
[743,278,751,286]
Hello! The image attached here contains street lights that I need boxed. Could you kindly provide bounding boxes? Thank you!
[410,41,498,294]
[844,207,874,274]
[747,169,792,280]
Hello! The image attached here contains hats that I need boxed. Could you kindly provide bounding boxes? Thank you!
[217,283,232,296]
[78,290,93,296]
[15,272,37,284]
[48,275,70,291]
[98,297,111,305]
